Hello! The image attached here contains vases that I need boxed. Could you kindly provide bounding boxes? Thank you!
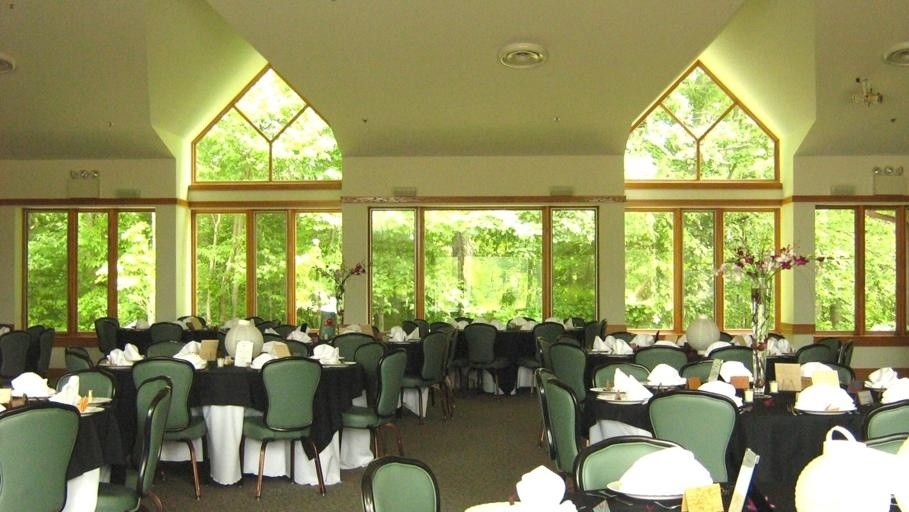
[751,277,771,340]
[335,285,345,325]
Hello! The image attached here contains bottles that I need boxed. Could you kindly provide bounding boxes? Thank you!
[319,318,336,347]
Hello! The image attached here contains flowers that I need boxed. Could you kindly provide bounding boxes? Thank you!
[706,234,816,341]
[312,260,366,321]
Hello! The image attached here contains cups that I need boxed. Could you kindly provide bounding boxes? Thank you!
[743,388,754,403]
[768,380,779,394]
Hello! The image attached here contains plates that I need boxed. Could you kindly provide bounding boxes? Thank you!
[454,325,584,331]
[464,501,519,509]
[387,335,420,345]
[100,355,357,367]
[798,409,855,416]
[765,351,797,360]
[589,382,678,405]
[0,395,112,416]
[587,349,633,359]
[607,481,682,502]
